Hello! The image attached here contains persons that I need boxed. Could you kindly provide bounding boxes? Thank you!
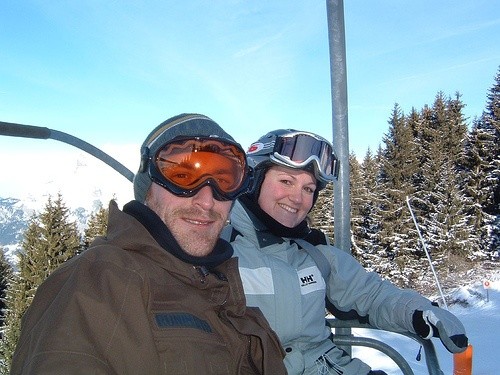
[10,114,289,375]
[218,129,469,375]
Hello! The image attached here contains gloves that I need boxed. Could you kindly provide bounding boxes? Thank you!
[411,301,468,354]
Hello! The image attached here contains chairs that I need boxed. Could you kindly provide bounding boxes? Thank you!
[326,319,442,375]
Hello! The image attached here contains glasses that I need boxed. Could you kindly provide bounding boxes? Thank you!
[138,135,248,201]
[246,131,338,181]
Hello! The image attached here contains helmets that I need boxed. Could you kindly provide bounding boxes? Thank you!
[132,112,249,205]
[243,128,328,199]
